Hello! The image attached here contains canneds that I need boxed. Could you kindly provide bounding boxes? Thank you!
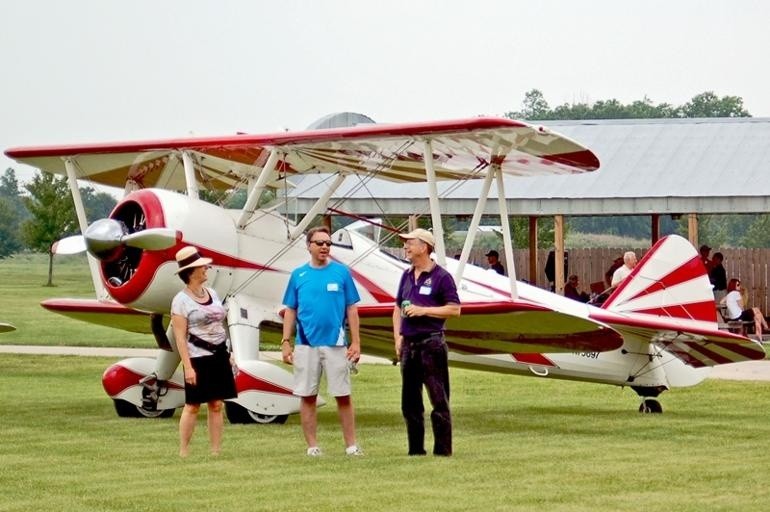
[400,299,411,319]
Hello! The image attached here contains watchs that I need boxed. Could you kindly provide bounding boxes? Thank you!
[281,337,291,345]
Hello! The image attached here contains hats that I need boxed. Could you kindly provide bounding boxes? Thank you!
[485,251,499,257]
[173,246,213,276]
[398,228,435,247]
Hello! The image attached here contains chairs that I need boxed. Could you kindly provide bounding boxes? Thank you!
[716,305,755,338]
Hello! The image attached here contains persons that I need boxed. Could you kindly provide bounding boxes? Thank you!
[720,279,769,343]
[455,254,460,260]
[484,250,504,275]
[390,226,463,460]
[169,244,241,461]
[612,251,637,288]
[565,275,590,303]
[700,245,727,316]
[277,226,365,460]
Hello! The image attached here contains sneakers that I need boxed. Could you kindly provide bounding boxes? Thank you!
[348,449,363,457]
[307,448,321,456]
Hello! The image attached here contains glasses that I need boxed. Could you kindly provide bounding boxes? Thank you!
[310,239,332,246]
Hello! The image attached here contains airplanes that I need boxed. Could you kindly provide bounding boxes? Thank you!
[3,118,766,425]
